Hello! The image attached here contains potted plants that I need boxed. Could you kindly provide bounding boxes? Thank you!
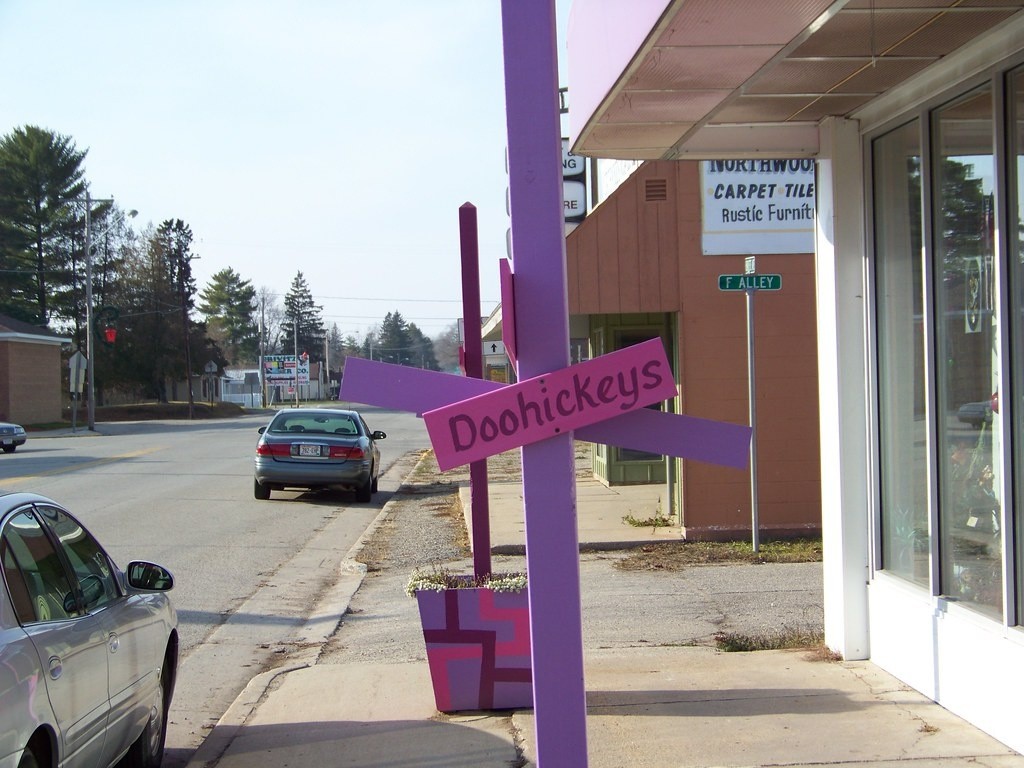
[406,557,534,711]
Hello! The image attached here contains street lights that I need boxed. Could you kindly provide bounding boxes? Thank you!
[87,209,140,432]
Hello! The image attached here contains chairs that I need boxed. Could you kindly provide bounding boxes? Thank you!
[289,425,305,431]
[335,428,351,433]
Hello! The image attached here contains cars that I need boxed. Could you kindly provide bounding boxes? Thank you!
[253,410,387,504]
[0,492,179,768]
[958,400,992,429]
[0,421,27,454]
